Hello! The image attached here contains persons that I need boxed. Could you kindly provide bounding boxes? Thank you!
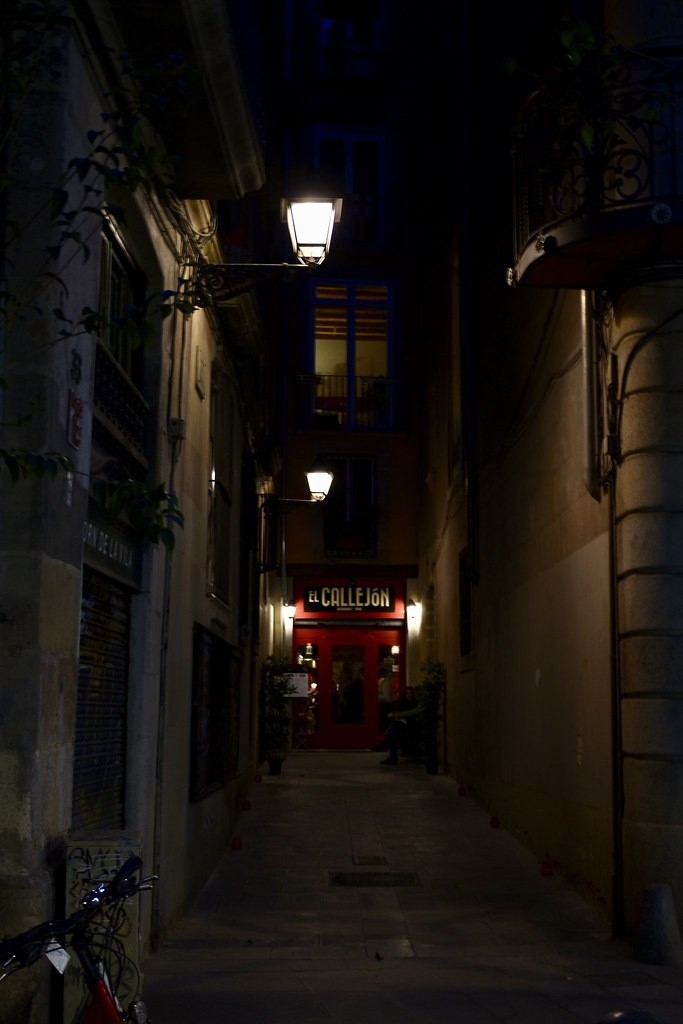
[376,671,394,702]
[336,661,365,721]
[380,685,430,768]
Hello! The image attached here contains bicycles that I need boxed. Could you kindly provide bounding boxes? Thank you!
[0,853,158,1024]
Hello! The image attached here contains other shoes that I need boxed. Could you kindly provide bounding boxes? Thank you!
[381,756,396,765]
[371,743,388,751]
